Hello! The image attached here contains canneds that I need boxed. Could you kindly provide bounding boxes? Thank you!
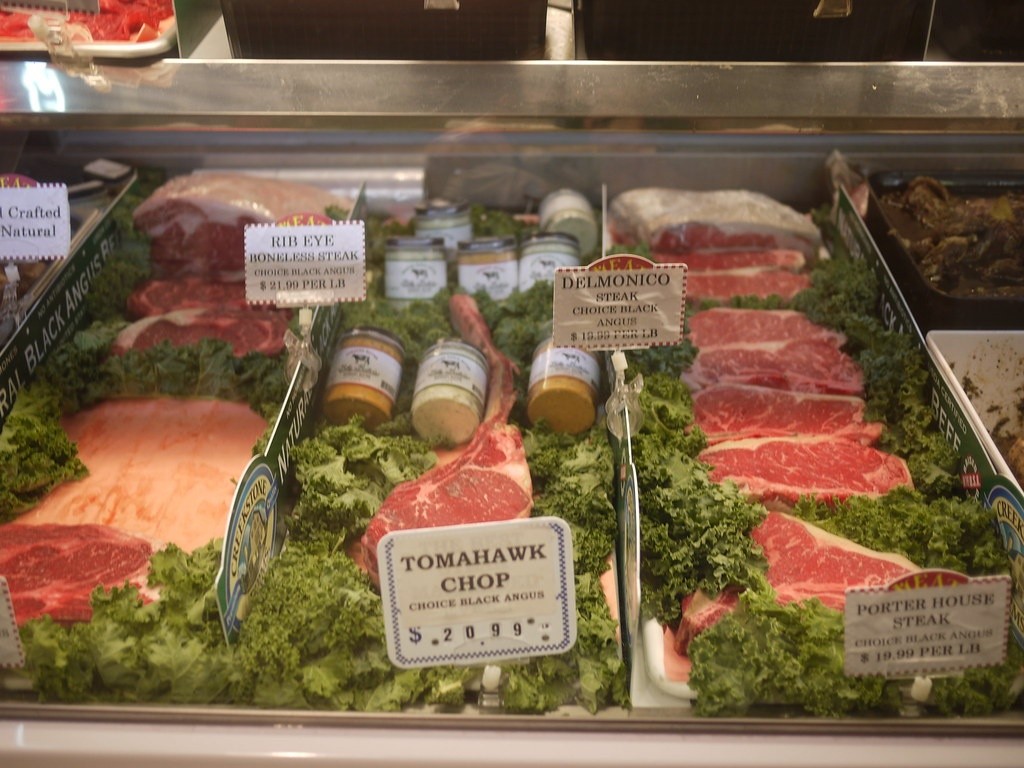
[382,233,447,307]
[323,323,404,430]
[455,233,520,301]
[536,185,599,260]
[412,335,490,445]
[412,194,475,268]
[516,231,583,292]
[525,335,600,436]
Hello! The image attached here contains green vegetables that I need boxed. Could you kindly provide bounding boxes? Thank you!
[1,173,1021,721]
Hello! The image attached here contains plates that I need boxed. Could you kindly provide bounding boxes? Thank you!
[0,15,174,57]
